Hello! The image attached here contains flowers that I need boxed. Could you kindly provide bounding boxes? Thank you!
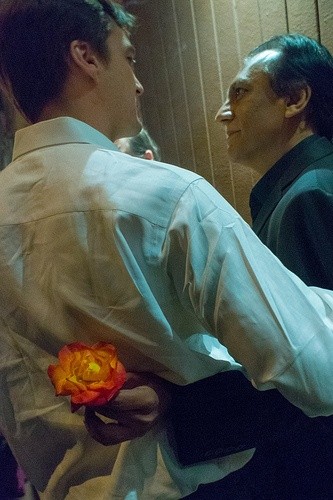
[47,340,126,413]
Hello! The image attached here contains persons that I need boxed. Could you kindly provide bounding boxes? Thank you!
[0,0,333,499]
[83,31,333,498]
